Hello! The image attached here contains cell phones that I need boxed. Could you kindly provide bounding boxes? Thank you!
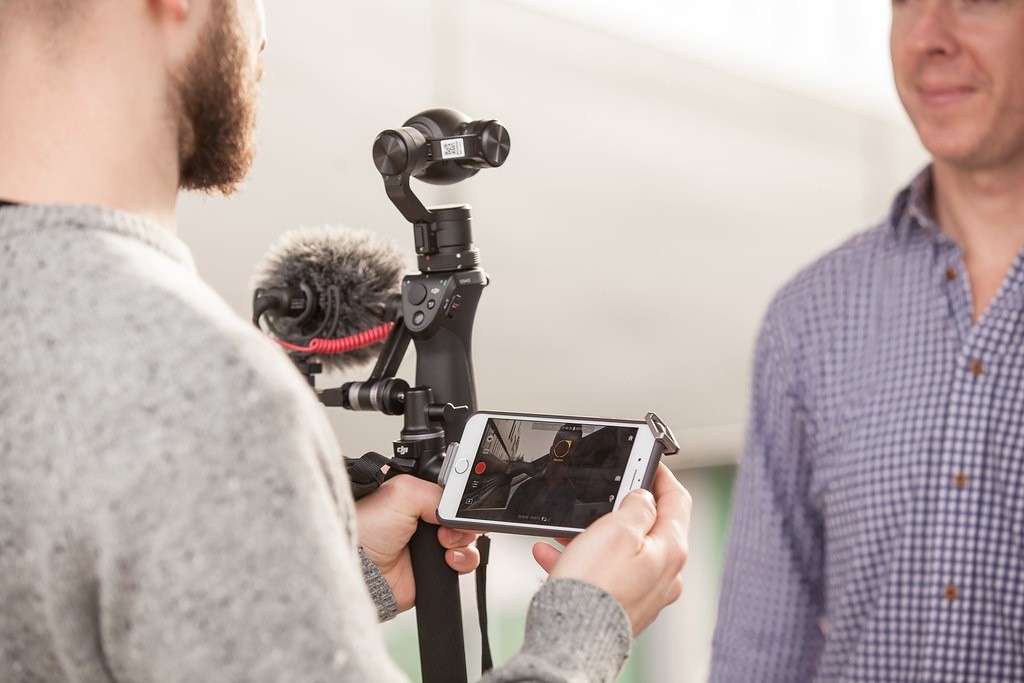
[435,410,665,539]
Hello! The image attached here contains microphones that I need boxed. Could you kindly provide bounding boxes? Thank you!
[251,223,408,371]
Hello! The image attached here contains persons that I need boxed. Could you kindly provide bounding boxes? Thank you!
[503,423,583,527]
[1,0,693,681]
[710,2,1024,681]
